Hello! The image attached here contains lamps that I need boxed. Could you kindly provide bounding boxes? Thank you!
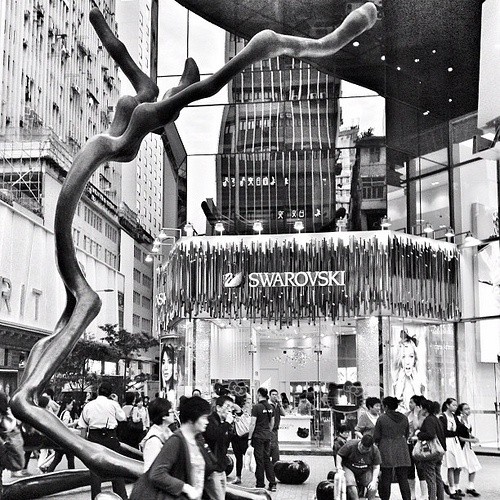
[445,227,456,244]
[183,222,193,237]
[145,227,181,262]
[335,217,347,232]
[293,218,305,234]
[380,215,392,230]
[423,222,436,240]
[252,219,264,235]
[214,220,225,235]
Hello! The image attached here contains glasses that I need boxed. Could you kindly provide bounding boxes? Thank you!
[224,405,235,410]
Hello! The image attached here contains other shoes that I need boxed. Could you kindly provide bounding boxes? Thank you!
[270,484,276,491]
[466,489,480,497]
[39,466,47,473]
[456,489,465,496]
[449,493,461,500]
[442,483,450,496]
[229,476,241,484]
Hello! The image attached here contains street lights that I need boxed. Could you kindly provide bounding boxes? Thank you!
[249,350,257,404]
[314,350,322,430]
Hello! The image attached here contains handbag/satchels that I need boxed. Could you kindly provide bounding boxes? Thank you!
[412,436,445,461]
[130,434,188,500]
[2,435,26,470]
[127,407,143,434]
[234,411,251,436]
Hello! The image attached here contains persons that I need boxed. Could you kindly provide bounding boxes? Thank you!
[159,342,177,406]
[393,330,426,405]
[0,381,482,500]
